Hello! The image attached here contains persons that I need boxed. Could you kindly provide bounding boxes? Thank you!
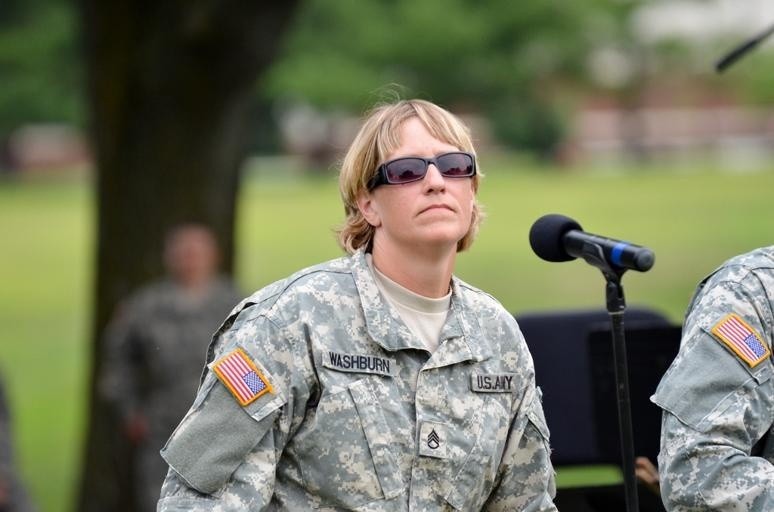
[96,225,246,512]
[656,244,774,512]
[156,99,559,512]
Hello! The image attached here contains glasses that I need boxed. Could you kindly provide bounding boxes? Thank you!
[367,150,475,192]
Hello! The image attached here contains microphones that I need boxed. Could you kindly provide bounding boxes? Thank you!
[528,212,655,273]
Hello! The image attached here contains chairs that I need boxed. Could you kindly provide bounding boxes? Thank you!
[516,305,667,509]
